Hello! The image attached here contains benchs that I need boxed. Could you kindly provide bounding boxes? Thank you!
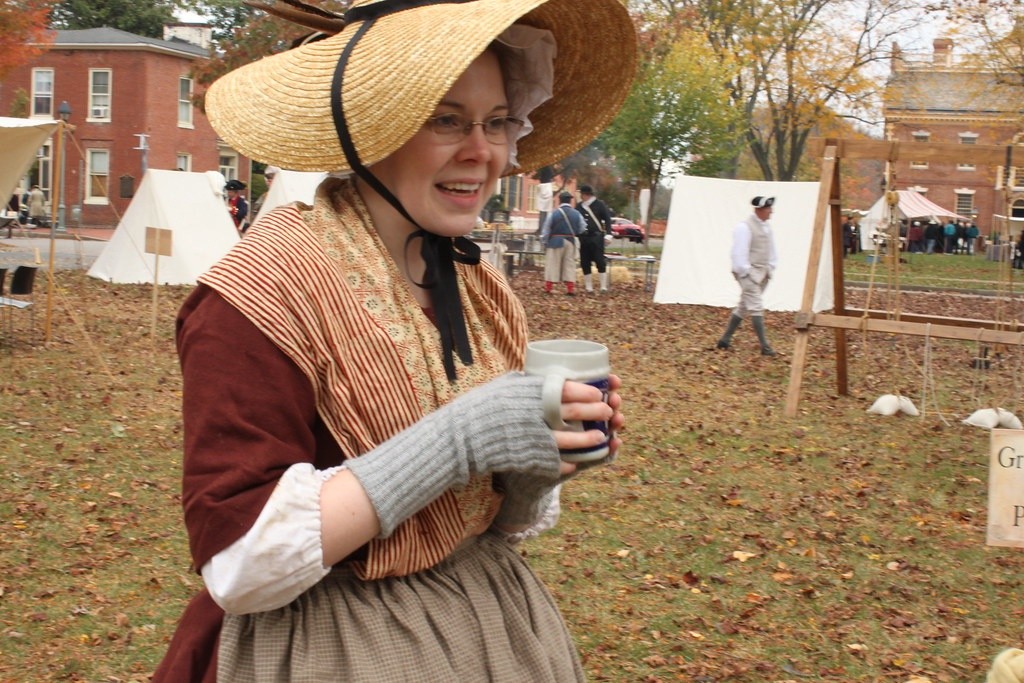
[480,250,546,267]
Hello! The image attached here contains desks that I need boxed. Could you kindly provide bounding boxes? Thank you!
[469,238,524,267]
[604,254,656,292]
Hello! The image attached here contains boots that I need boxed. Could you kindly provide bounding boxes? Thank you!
[752,315,776,357]
[716,312,742,351]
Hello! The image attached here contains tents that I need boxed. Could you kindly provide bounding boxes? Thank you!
[859,190,968,251]
[85,168,245,286]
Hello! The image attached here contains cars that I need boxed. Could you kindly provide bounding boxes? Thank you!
[603,217,642,243]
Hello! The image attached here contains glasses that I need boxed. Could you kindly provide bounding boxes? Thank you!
[423,113,522,149]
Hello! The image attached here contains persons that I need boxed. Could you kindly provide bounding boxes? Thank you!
[540,191,587,294]
[254,165,282,215]
[842,217,856,256]
[900,219,980,255]
[156,1,639,682]
[26,184,46,226]
[1013,229,1024,269]
[573,186,612,292]
[716,195,780,359]
[223,179,250,240]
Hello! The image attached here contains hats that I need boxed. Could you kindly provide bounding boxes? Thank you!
[558,192,573,198]
[579,185,592,194]
[913,221,920,226]
[204,0,635,298]
[928,219,937,224]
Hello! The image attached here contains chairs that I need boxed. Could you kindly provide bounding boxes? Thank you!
[0,266,37,349]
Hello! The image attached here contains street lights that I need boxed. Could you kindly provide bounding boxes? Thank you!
[55,101,72,234]
[630,177,637,220]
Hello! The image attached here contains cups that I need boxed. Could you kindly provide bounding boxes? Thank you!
[523,340,612,463]
